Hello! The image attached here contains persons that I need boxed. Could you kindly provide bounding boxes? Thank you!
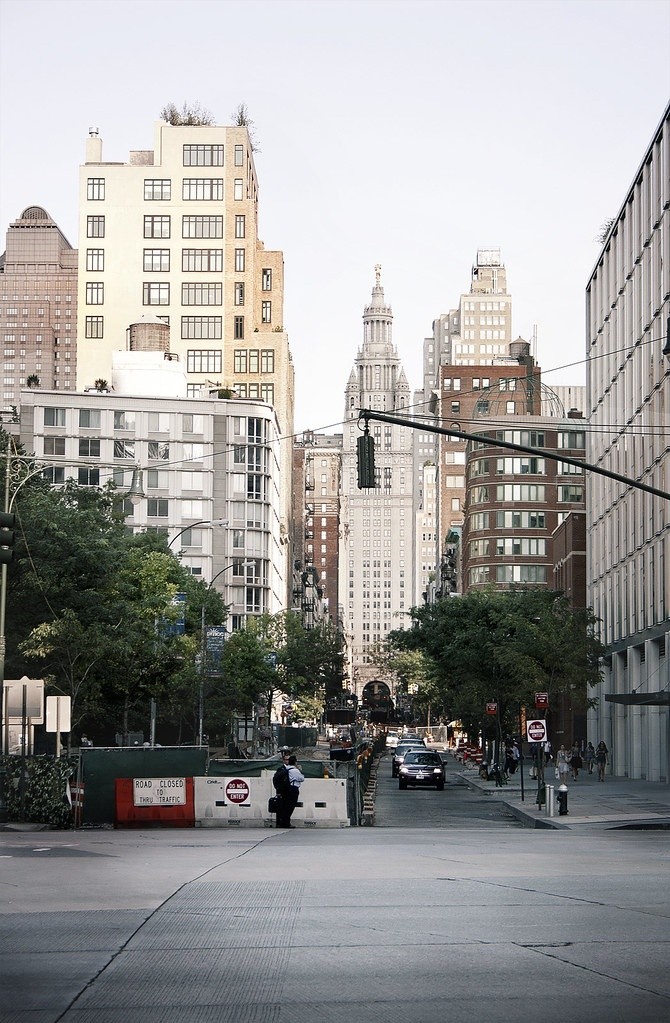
[595,741,610,782]
[504,745,519,774]
[566,742,584,780]
[228,736,242,759]
[541,741,552,768]
[556,744,569,785]
[584,742,595,774]
[258,724,270,747]
[276,754,304,828]
[529,744,538,762]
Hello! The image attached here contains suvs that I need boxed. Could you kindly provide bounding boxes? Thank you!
[398,749,447,790]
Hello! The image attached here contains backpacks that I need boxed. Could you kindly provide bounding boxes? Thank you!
[273,765,296,793]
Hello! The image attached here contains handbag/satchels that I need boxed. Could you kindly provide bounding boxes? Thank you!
[555,766,560,779]
[594,757,598,764]
[565,750,569,762]
[269,795,284,813]
[529,763,534,775]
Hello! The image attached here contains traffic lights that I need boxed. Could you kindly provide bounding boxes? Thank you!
[0,511,15,564]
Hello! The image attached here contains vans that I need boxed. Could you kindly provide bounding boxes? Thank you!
[391,744,426,779]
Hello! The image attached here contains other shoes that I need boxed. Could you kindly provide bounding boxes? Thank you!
[276,823,296,828]
[531,776,537,780]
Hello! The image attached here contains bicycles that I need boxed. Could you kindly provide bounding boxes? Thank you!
[492,762,505,787]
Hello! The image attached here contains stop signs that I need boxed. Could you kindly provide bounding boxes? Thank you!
[526,719,547,743]
[224,778,250,804]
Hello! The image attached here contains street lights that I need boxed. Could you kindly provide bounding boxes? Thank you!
[199,560,257,745]
[0,432,145,754]
[150,519,230,749]
[262,607,301,648]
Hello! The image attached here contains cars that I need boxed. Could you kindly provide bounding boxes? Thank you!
[398,733,419,739]
[397,739,425,747]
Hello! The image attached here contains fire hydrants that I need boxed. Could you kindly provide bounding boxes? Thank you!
[557,784,569,815]
